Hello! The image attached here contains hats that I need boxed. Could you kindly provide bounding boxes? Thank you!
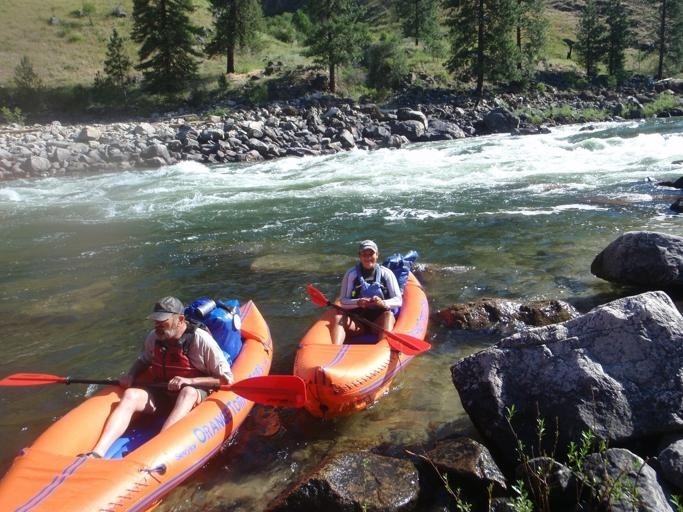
[145,296,184,322]
[359,240,378,253]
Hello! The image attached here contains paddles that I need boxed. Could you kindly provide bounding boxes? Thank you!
[308,283,432,355]
[0,373,308,408]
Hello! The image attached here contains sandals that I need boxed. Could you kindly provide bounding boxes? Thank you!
[77,452,101,458]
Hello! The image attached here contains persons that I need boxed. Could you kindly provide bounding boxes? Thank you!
[331,239,402,344]
[76,296,234,461]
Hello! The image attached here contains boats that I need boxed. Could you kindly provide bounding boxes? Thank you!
[0,298,273,512]
[293,269,430,419]
